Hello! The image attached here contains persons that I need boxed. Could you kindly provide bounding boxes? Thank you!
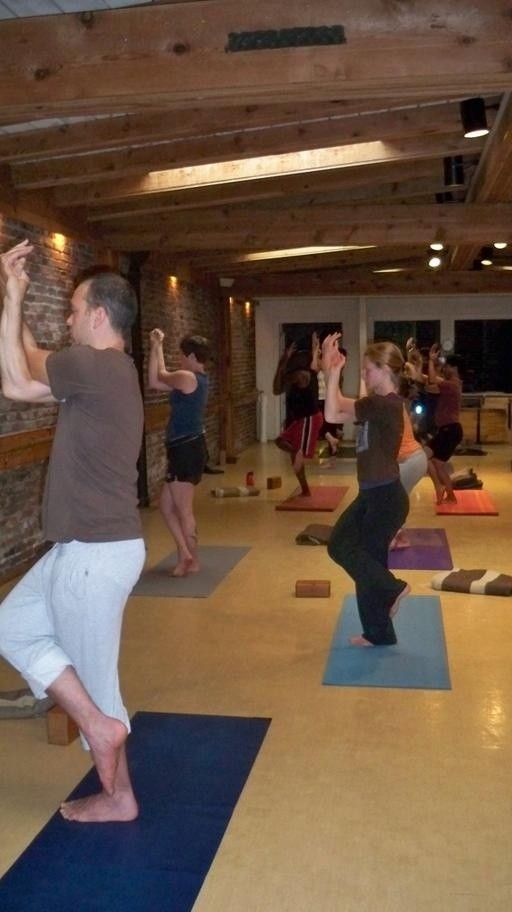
[404,338,462,505]
[388,405,427,551]
[274,332,347,497]
[0,237,145,822]
[322,332,411,647]
[146,328,211,578]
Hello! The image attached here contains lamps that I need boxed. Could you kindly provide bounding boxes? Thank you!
[460,97,490,140]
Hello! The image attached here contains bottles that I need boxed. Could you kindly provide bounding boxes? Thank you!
[246,471,255,485]
